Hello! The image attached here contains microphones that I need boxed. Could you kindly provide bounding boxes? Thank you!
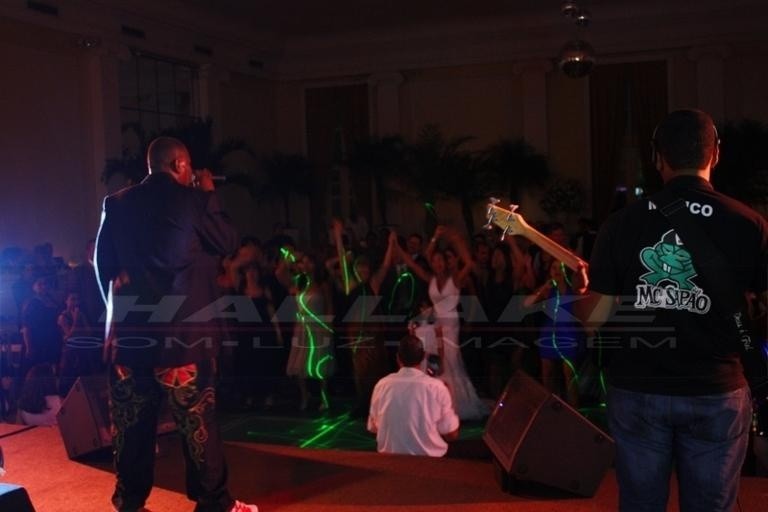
[192,173,227,183]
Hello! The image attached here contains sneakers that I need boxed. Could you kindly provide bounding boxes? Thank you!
[229,498,258,512]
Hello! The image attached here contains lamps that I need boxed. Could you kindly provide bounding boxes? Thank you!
[550,1,604,86]
